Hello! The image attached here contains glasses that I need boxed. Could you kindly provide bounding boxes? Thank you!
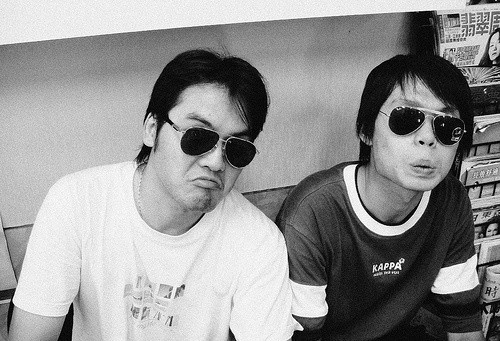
[166,118,260,169]
[379,105,467,146]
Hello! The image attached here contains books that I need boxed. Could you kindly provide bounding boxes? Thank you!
[457,111,500,341]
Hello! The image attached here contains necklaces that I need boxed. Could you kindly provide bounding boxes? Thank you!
[138,166,146,220]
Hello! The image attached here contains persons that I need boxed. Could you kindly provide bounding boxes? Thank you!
[9,48,292,341]
[276,54,485,341]
[445,49,454,63]
[478,28,500,66]
[480,216,500,237]
